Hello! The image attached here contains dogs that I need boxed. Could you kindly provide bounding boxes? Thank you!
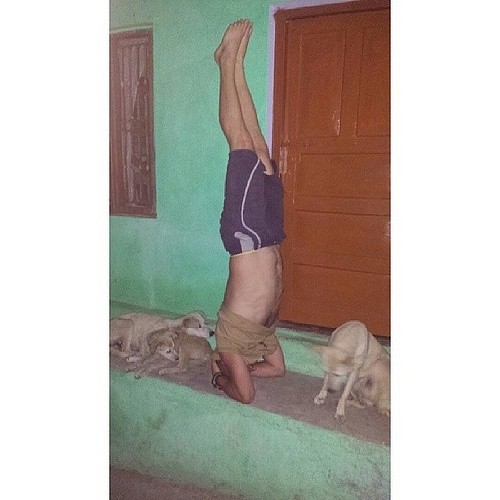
[109,312,214,359]
[125,328,213,379]
[309,320,390,424]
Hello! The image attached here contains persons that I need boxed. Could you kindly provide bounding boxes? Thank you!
[211,15,291,407]
